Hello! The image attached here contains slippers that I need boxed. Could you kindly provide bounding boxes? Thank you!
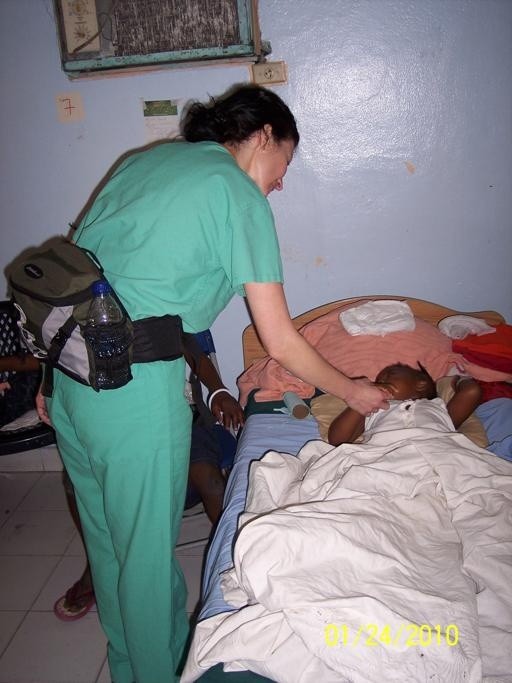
[54,581,96,621]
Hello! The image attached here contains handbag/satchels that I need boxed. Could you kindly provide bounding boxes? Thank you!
[3,235,134,392]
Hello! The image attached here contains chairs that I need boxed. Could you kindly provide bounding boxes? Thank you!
[0,300,67,456]
[172,328,239,554]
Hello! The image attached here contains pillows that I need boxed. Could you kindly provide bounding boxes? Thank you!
[310,376,489,448]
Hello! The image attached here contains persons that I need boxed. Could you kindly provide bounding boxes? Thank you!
[328,363,483,447]
[35,84,389,683]
[0,333,246,622]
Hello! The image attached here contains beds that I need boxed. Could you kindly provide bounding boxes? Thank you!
[181,295,512,683]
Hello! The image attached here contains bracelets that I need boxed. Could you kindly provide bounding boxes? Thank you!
[208,389,233,412]
[455,378,470,392]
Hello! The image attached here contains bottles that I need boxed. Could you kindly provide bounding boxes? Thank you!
[85,281,130,390]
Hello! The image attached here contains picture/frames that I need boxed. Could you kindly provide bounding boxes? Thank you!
[52,0,273,81]
